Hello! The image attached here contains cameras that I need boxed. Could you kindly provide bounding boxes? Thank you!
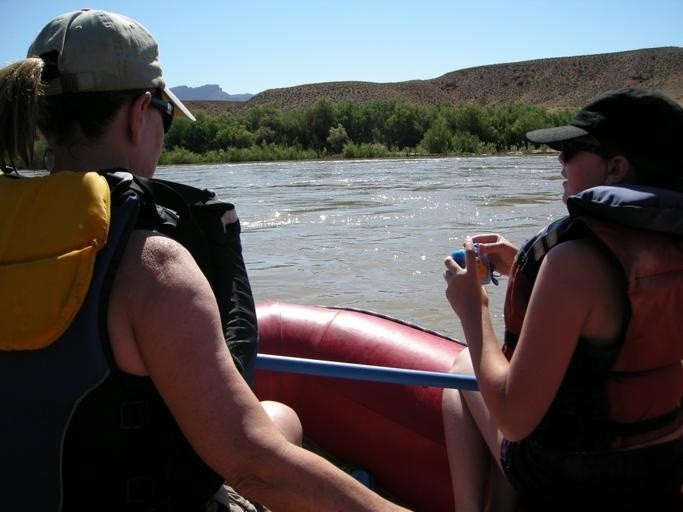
[453,244,492,284]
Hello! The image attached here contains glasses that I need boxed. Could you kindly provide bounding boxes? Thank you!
[148,98,174,135]
[560,140,603,164]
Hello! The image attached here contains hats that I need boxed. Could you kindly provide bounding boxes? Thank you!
[26,7,197,124]
[525,86,683,181]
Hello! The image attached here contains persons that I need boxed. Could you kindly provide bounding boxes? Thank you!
[442,88,683,512]
[0,7,416,512]
